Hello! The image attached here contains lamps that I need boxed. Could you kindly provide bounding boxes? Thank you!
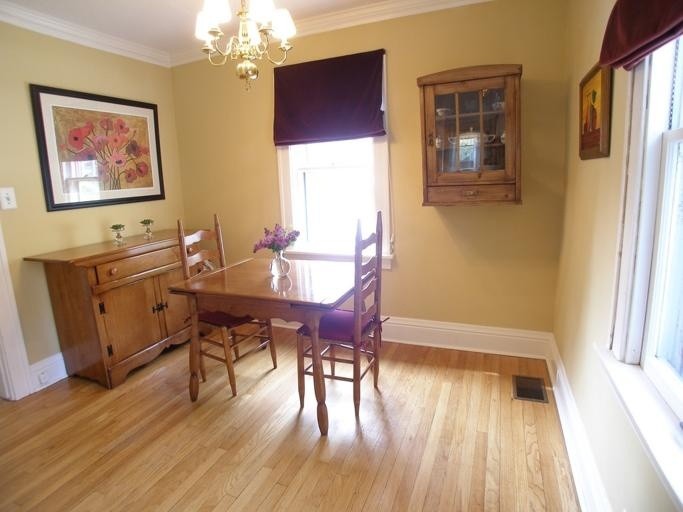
[193,0,299,92]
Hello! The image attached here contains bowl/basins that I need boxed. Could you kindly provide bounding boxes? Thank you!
[492,102,504,110]
[448,127,496,147]
[436,108,451,116]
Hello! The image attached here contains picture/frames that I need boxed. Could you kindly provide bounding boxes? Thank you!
[576,59,614,161]
[28,79,166,213]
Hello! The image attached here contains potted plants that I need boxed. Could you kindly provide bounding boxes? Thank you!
[139,219,156,239]
[109,223,127,243]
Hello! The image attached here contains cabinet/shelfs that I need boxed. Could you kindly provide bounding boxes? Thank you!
[21,230,211,389]
[416,62,524,206]
